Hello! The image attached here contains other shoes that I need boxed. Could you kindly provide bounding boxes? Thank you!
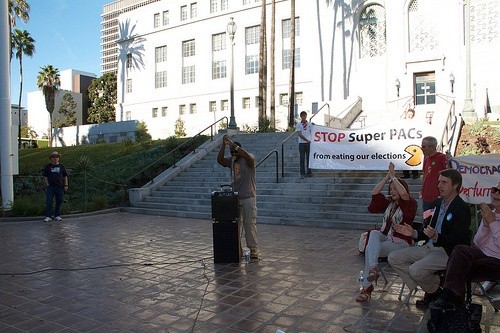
[416,286,444,308]
[301,172,305,175]
[251,250,258,259]
[307,172,312,177]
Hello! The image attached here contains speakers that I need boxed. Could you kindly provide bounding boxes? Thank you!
[211,191,240,220]
[212,222,242,263]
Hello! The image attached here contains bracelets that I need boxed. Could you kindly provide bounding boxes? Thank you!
[64,185,68,187]
[383,179,388,182]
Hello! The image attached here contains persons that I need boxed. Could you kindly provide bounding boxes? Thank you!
[43,151,69,222]
[217,134,259,259]
[432,182,500,309]
[422,137,452,229]
[356,162,418,304]
[400,109,419,179]
[387,169,471,311]
[296,111,314,179]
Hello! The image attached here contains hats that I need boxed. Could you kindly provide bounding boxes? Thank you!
[49,151,60,160]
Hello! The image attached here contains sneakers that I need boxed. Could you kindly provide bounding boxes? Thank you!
[54,216,62,221]
[44,217,52,222]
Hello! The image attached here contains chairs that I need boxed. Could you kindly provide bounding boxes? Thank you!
[375,229,418,300]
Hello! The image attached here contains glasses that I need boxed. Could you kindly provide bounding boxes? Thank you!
[51,156,59,158]
[491,187,500,194]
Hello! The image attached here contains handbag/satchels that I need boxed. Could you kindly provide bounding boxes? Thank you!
[427,302,482,333]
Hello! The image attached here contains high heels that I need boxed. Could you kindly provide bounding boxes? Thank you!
[356,282,374,302]
[367,268,380,286]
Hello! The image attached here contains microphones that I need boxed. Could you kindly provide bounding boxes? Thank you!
[232,156,237,161]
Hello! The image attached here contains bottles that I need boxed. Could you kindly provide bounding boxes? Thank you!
[359,271,365,290]
[244,251,251,260]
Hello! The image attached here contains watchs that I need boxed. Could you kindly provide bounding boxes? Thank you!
[392,177,397,181]
[235,146,240,150]
